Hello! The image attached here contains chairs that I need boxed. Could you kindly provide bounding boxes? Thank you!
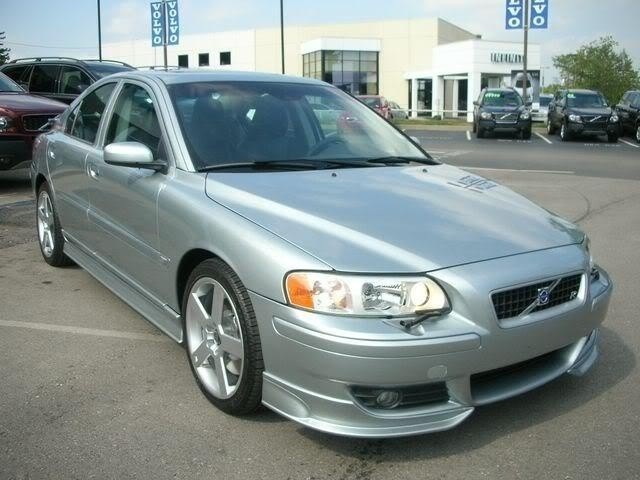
[64,75,82,95]
[147,98,303,165]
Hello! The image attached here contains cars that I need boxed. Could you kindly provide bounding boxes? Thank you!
[336,95,394,134]
[308,102,341,124]
[388,100,409,121]
[31,66,612,439]
[0,72,86,172]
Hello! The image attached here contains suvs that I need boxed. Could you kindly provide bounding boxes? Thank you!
[0,56,152,118]
[613,89,640,144]
[544,82,623,148]
[472,86,534,141]
